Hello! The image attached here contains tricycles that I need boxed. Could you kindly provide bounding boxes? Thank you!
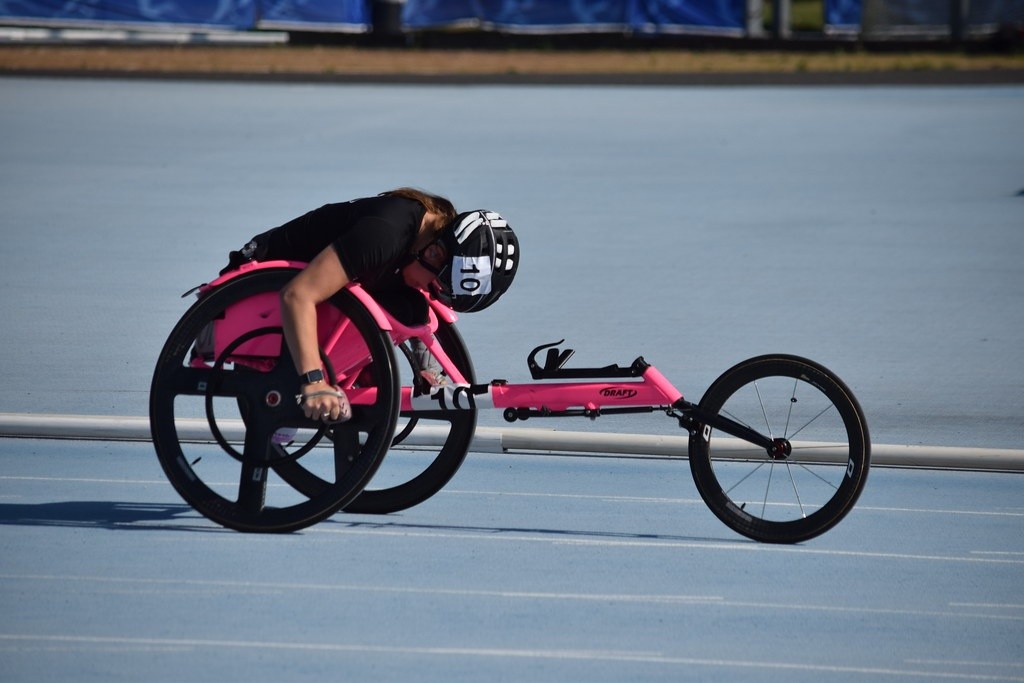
[148,262,871,544]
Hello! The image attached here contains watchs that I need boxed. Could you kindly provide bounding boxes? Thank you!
[299,370,326,386]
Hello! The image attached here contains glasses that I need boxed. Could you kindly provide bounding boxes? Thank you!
[427,280,451,308]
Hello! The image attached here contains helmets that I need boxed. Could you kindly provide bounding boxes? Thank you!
[416,209,520,313]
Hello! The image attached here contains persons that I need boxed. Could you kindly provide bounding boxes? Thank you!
[219,187,520,421]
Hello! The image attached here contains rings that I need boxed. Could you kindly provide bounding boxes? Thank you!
[324,413,330,416]
